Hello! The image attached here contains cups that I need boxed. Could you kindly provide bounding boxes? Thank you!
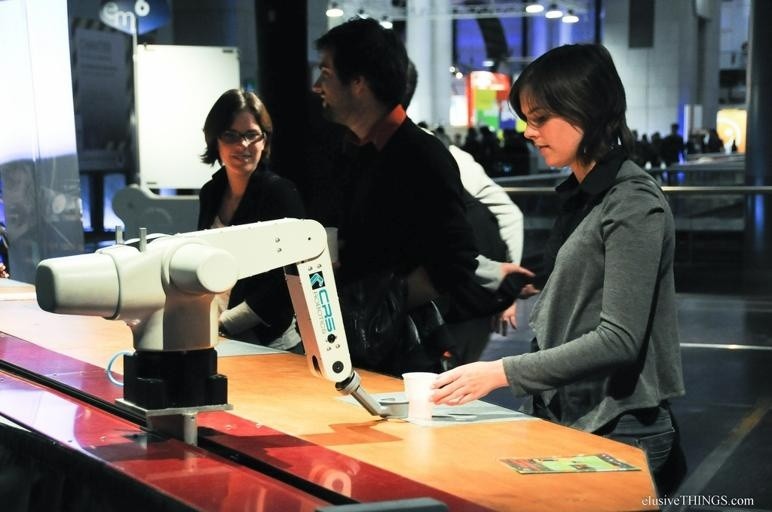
[402,372,439,421]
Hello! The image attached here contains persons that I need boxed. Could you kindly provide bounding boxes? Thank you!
[629,123,726,180]
[197,89,308,348]
[429,42,687,476]
[418,122,531,179]
[289,18,469,376]
[442,126,540,370]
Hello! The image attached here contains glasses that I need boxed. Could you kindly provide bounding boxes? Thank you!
[220,130,266,144]
